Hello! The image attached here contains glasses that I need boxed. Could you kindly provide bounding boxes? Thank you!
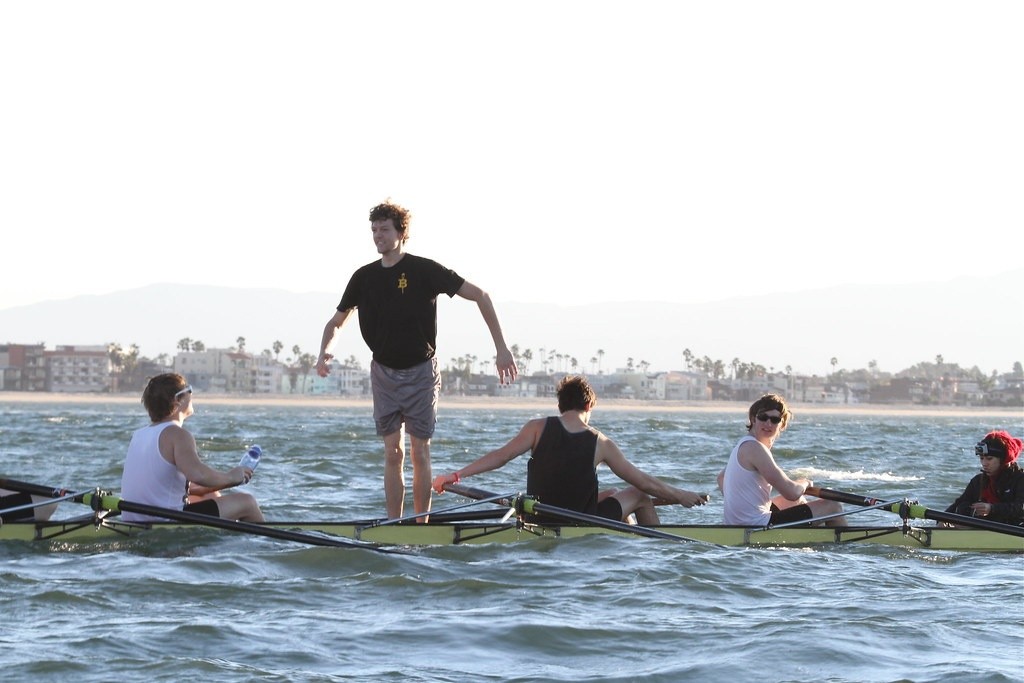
[174,385,192,399]
[755,412,781,424]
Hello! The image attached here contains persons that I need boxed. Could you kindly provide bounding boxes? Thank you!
[432,374,706,527]
[936,431,1024,538]
[717,392,851,526]
[117,371,268,524]
[314,203,517,527]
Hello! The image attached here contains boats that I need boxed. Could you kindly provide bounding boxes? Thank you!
[1,510,1024,553]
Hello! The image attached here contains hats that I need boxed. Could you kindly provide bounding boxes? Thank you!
[985,431,1023,467]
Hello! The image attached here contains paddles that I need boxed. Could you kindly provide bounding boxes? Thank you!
[805,487,1024,537]
[442,481,701,546]
[0,472,372,553]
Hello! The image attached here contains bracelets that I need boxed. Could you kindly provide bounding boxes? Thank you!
[452,471,459,483]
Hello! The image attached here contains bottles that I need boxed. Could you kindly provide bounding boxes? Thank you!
[239,444,262,482]
